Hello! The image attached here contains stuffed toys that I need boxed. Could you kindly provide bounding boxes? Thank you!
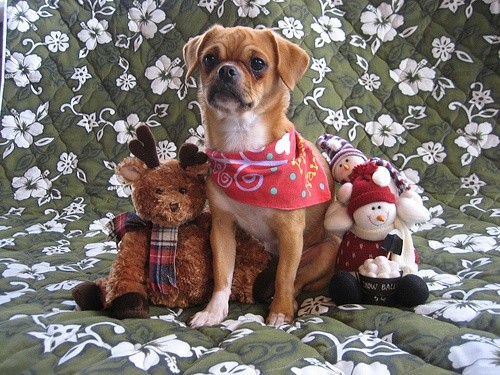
[328,163,429,306]
[72,125,274,318]
[314,133,424,233]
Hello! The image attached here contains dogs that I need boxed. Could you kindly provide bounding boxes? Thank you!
[183,23,342,328]
